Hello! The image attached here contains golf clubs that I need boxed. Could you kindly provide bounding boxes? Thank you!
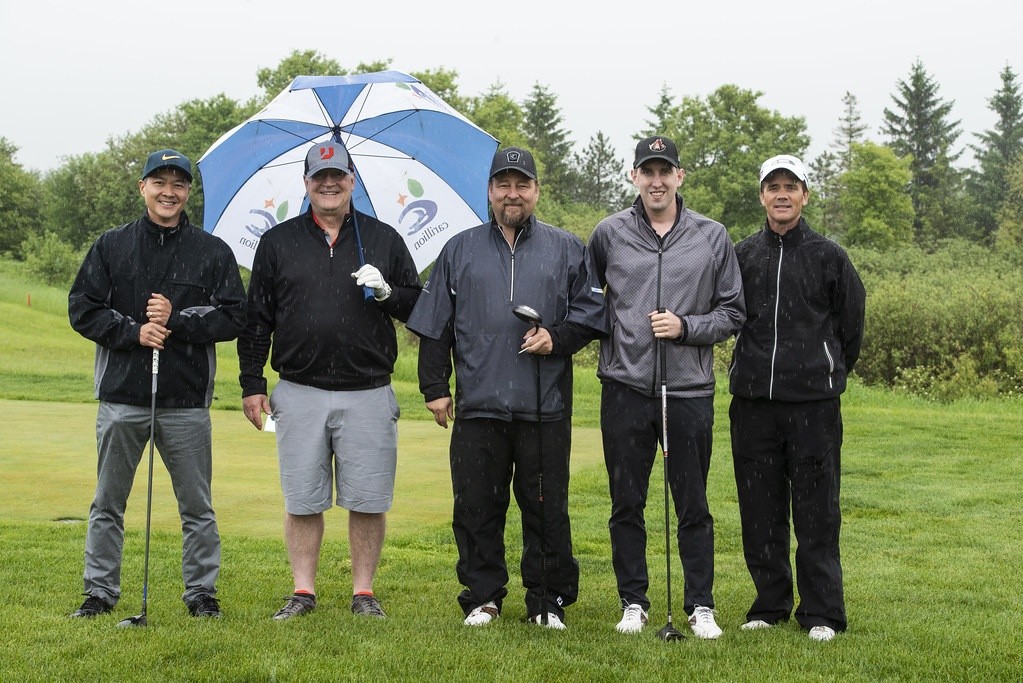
[655,307,689,641]
[512,304,549,626]
[115,349,160,628]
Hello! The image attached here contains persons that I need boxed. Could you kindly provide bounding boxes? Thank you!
[729,155,867,640]
[587,135,747,639]
[406,147,611,631]
[237,142,422,621]
[69,150,249,620]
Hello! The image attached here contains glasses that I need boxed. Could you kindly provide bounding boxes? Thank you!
[312,171,346,179]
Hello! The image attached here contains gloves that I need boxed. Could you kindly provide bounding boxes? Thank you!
[351,264,392,302]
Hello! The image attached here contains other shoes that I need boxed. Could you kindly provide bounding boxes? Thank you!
[808,626,835,641]
[741,620,772,630]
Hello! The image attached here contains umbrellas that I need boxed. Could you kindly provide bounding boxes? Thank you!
[195,69,501,303]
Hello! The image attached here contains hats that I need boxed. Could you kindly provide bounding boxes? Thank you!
[304,140,354,178]
[760,155,808,190]
[489,147,536,179]
[633,136,678,169]
[142,149,192,183]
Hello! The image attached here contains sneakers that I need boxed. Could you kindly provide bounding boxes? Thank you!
[528,613,566,630]
[351,591,386,618]
[615,598,648,635]
[70,593,113,619]
[189,595,223,618]
[462,601,499,627]
[273,590,315,619]
[688,604,722,640]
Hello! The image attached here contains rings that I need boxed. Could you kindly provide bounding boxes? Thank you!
[150,312,153,318]
